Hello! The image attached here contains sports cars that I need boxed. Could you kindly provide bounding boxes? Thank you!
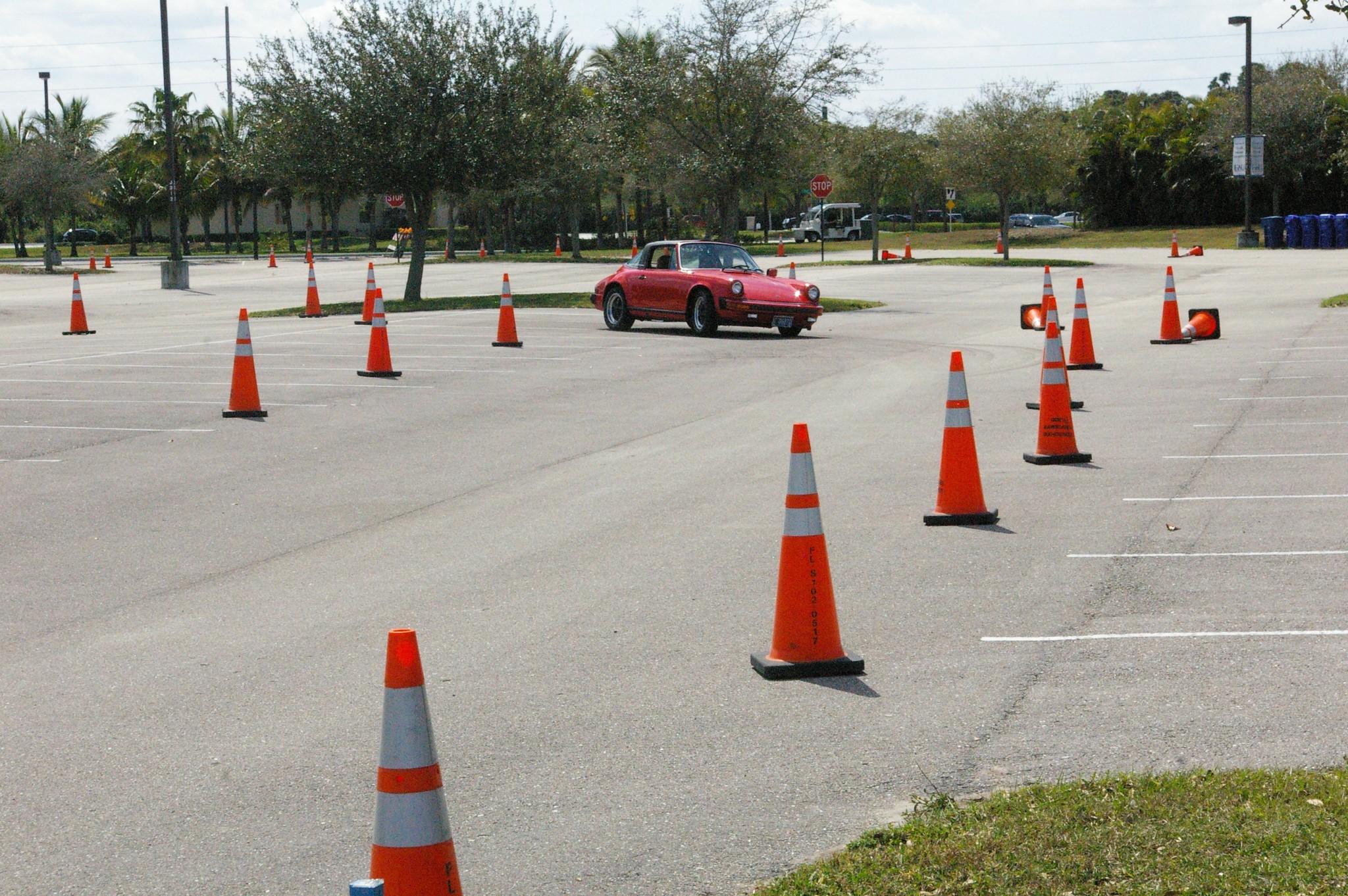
[588,239,824,338]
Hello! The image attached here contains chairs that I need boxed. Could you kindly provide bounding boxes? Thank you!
[657,256,676,269]
[698,253,723,268]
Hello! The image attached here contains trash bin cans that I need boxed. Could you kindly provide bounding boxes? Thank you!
[1260,216,1285,249]
[1284,214,1302,249]
[1317,213,1336,249]
[43,250,63,265]
[1335,214,1348,249]
[1299,215,1318,249]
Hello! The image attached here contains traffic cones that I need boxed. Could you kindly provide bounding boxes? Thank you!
[489,276,528,347]
[1167,230,1205,258]
[352,262,411,380]
[441,238,449,260]
[266,244,278,267]
[883,237,915,259]
[923,270,1221,526]
[369,627,465,896]
[776,232,787,259]
[62,245,114,336]
[749,424,876,683]
[630,235,638,260]
[662,235,671,258]
[788,259,798,281]
[478,238,486,257]
[222,307,270,418]
[553,236,564,257]
[300,239,329,318]
[993,228,1005,254]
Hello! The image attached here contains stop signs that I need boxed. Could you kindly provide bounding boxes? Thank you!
[385,190,405,207]
[809,174,833,198]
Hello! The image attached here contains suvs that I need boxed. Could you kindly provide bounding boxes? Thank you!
[1008,212,1083,230]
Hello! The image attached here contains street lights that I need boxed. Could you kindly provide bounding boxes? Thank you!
[1224,14,1261,246]
[37,70,63,268]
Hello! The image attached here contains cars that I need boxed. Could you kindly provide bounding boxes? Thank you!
[781,203,964,241]
[62,228,98,243]
